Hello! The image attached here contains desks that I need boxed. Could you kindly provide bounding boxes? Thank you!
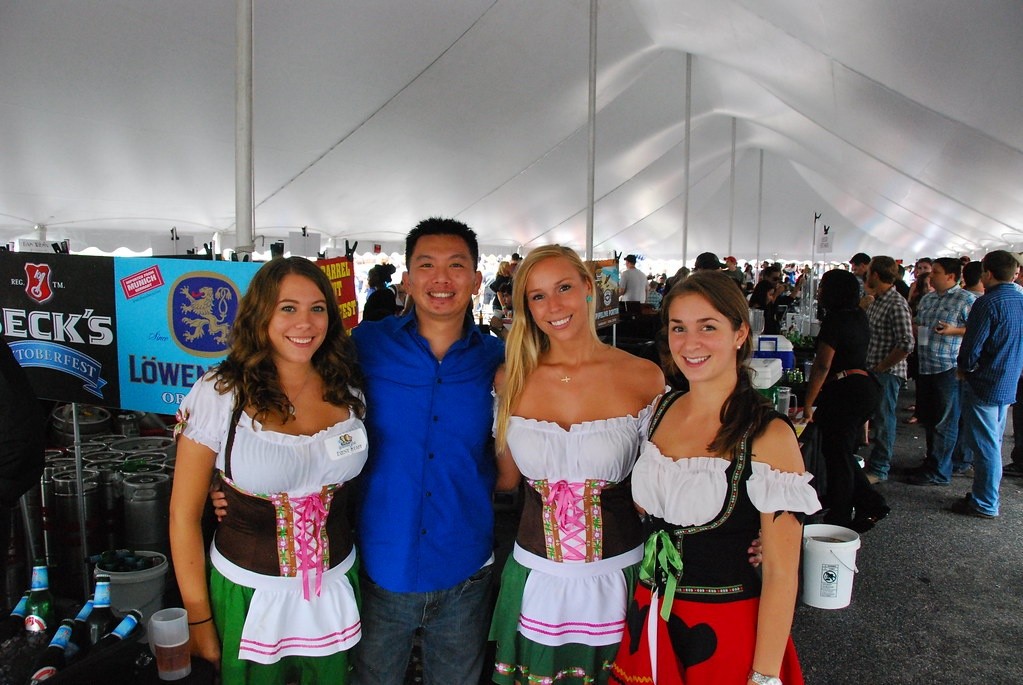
[789,406,820,491]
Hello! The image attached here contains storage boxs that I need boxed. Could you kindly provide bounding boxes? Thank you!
[0,592,148,685]
[744,358,783,412]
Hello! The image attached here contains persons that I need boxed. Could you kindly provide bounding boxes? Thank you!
[952,250,1023,518]
[0,331,48,639]
[850,251,1023,486]
[798,269,892,534]
[610,267,822,685]
[495,245,764,685]
[210,219,518,685]
[363,262,396,324]
[168,254,371,685]
[490,252,820,341]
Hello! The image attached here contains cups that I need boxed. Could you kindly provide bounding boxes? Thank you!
[147,607,191,680]
[917,325,930,346]
[776,386,797,417]
[493,309,502,319]
[507,310,512,318]
[787,309,820,338]
[776,283,788,296]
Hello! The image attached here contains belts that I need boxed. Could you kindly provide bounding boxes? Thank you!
[833,369,869,380]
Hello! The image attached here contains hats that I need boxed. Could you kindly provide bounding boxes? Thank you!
[724,256,737,263]
[696,252,727,269]
[624,254,636,264]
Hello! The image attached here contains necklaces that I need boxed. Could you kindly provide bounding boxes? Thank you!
[539,347,598,384]
[274,368,312,414]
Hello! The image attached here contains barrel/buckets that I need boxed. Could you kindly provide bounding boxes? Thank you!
[94,550,169,644]
[38,403,176,569]
[853,453,865,472]
[800,523,861,610]
[748,309,765,335]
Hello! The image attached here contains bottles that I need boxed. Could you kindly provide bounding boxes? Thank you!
[479,310,483,325]
[59,594,95,671]
[25,618,76,685]
[785,331,817,349]
[25,558,55,649]
[85,573,118,646]
[84,548,164,573]
[0,589,31,644]
[85,609,143,659]
[781,367,804,383]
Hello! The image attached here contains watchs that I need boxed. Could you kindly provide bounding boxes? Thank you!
[747,669,783,685]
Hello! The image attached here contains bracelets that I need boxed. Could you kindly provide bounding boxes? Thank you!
[187,616,212,625]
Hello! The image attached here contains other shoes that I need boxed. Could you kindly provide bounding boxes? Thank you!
[864,470,880,484]
[905,467,951,486]
[823,511,836,523]
[948,493,994,518]
[854,504,891,534]
[905,403,916,410]
[1003,462,1023,477]
[907,416,919,423]
[952,467,975,478]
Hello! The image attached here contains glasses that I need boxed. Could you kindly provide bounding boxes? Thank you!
[768,277,780,281]
